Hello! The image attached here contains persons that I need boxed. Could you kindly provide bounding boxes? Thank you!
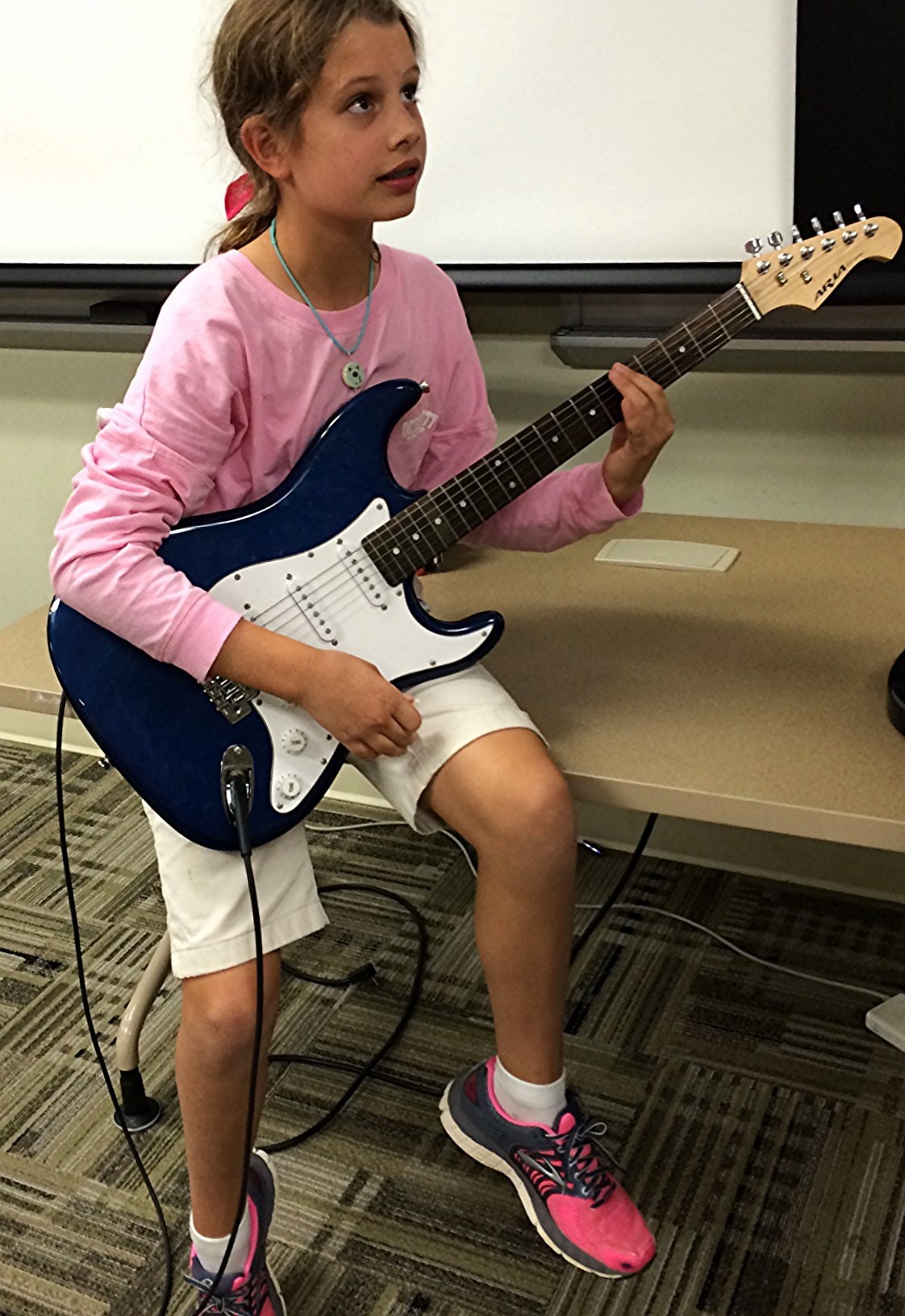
[47,0,678,1316]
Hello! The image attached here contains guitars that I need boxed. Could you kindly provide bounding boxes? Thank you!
[45,204,903,852]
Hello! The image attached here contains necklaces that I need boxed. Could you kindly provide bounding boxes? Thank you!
[270,216,374,389]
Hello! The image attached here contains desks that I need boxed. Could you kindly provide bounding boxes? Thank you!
[0,484,905,1133]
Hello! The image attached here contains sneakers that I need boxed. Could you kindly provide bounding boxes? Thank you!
[183,1147,287,1316]
[437,1057,659,1279]
